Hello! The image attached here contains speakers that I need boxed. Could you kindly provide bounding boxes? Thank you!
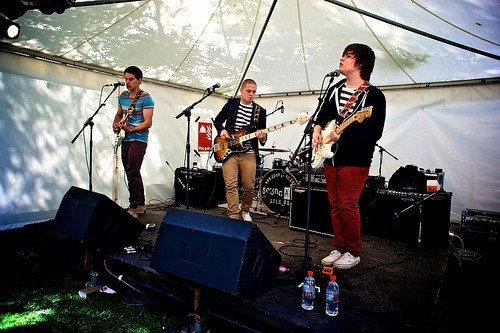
[149,207,282,301]
[367,187,453,248]
[290,184,365,237]
[175,167,218,210]
[55,185,146,256]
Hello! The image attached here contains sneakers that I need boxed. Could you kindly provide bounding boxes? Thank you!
[321,250,342,265]
[333,252,361,269]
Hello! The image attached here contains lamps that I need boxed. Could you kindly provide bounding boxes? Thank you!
[0,16,20,40]
[0,0,28,20]
[30,0,76,15]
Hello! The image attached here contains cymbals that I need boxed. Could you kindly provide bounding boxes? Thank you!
[257,147,291,153]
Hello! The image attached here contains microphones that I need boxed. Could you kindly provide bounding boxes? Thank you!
[281,102,284,113]
[105,82,125,86]
[326,70,340,77]
[207,83,220,90]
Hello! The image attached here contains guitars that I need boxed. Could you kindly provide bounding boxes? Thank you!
[113,106,135,154]
[310,107,373,169]
[213,114,311,163]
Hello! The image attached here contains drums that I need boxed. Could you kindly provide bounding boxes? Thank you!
[259,168,306,219]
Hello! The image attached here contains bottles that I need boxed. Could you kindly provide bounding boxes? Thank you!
[90,271,98,286]
[193,154,197,169]
[194,313,201,333]
[326,275,340,316]
[302,271,316,310]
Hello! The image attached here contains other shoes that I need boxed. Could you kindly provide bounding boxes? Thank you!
[127,208,138,218]
[136,205,148,214]
[241,211,252,221]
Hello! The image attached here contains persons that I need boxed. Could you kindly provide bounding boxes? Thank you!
[113,66,154,218]
[312,44,386,269]
[213,79,267,222]
